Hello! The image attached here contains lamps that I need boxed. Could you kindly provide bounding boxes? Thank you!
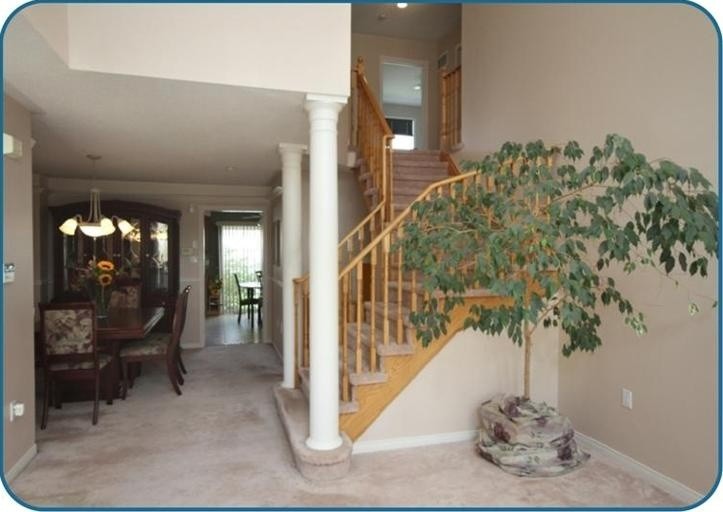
[56,152,138,240]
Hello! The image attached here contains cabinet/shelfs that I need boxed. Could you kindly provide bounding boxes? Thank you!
[49,198,182,333]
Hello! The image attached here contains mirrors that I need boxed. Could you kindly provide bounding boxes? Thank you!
[62,218,169,299]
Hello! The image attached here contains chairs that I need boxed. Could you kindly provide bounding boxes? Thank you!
[233,271,263,329]
[36,284,189,430]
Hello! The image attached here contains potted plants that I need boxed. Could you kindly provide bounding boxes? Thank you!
[388,132,718,484]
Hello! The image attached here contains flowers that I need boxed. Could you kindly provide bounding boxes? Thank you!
[86,260,128,313]
